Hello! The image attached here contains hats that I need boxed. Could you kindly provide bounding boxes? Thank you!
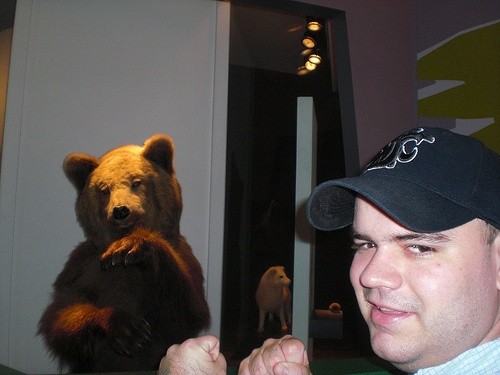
[306,127,500,234]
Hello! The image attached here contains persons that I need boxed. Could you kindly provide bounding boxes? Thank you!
[157,125,500,375]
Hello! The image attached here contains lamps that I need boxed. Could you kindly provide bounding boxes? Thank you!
[294,15,326,76]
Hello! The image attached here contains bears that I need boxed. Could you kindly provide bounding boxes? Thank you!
[34,133,212,375]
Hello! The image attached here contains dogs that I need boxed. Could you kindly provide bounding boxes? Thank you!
[257,266,292,336]
